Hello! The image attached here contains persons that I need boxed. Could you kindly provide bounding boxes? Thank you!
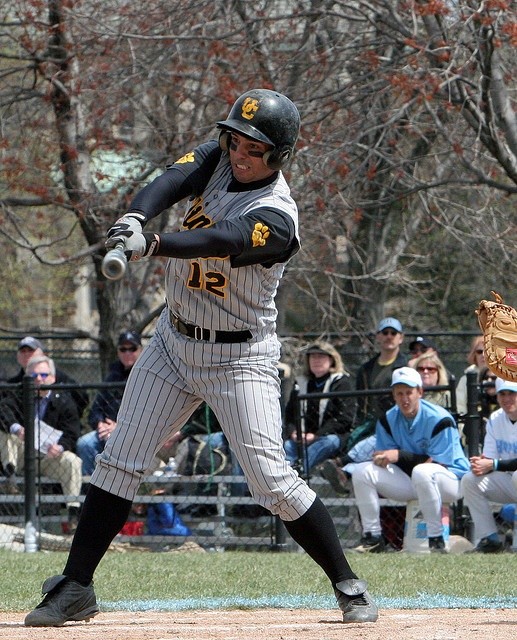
[281,339,358,483]
[349,365,471,554]
[454,334,494,420]
[165,426,250,518]
[4,335,88,417]
[358,316,407,438]
[408,348,452,416]
[461,368,517,556]
[76,334,187,482]
[2,354,85,538]
[24,89,377,626]
[403,330,431,364]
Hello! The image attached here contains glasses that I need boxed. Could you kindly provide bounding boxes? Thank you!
[474,349,483,354]
[381,330,397,336]
[411,347,426,354]
[29,372,49,377]
[416,367,438,374]
[118,346,138,352]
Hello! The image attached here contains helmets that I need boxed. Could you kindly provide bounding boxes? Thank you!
[214,88,301,170]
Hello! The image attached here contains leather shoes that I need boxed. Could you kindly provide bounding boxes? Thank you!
[133,505,147,515]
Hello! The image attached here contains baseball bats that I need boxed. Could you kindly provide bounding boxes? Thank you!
[101,241,128,282]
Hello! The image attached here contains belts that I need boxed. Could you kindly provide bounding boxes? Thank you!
[166,309,253,344]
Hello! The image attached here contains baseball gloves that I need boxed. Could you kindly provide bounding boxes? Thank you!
[476,290,517,382]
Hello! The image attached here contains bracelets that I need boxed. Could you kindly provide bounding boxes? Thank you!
[489,452,502,473]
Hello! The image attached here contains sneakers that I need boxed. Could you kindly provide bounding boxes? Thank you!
[464,533,506,554]
[429,537,446,554]
[342,532,384,553]
[334,579,376,623]
[233,505,258,518]
[0,478,22,496]
[317,456,347,492]
[191,503,217,517]
[65,514,78,535]
[24,574,98,625]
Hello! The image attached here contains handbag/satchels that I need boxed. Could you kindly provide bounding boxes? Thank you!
[174,433,231,479]
[145,488,192,537]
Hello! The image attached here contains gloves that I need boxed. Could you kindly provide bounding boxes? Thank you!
[105,230,159,261]
[105,212,146,236]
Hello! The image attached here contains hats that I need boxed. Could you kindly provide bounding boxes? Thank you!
[408,336,433,349]
[16,336,41,350]
[376,317,402,334]
[389,367,422,388]
[306,345,332,358]
[116,332,141,345]
[494,377,517,394]
